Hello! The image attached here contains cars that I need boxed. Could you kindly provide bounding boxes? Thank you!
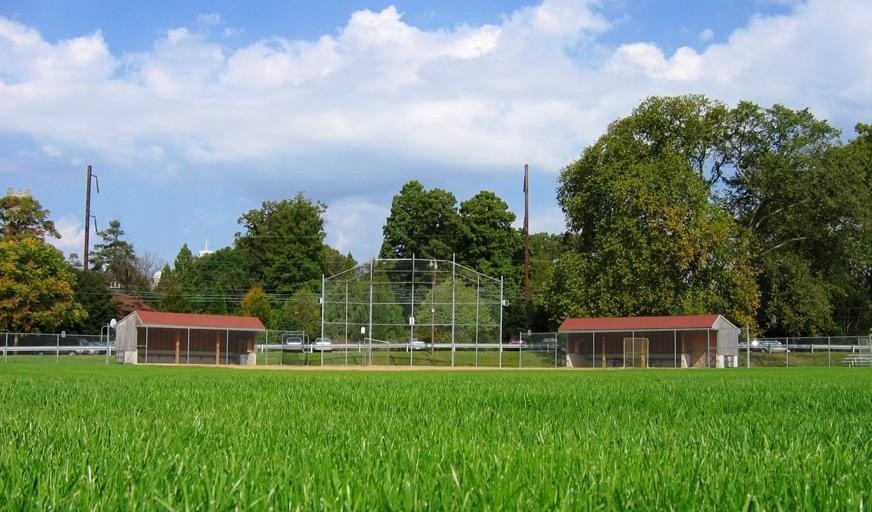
[740,338,790,354]
[84,340,118,354]
[408,335,426,349]
[285,335,333,353]
[508,335,529,351]
[542,337,562,351]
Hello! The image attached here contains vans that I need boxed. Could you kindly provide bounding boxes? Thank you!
[31,334,88,356]
